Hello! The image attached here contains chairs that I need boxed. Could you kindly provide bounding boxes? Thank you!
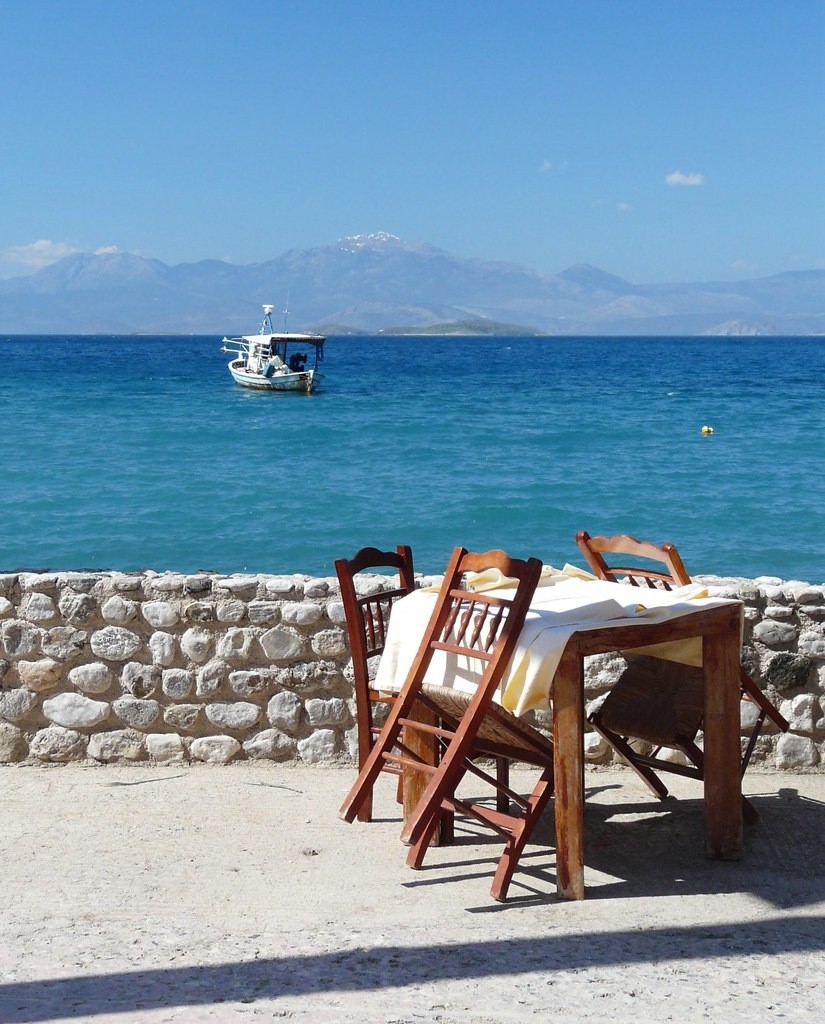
[338,547,554,901]
[575,533,790,825]
[334,545,511,837]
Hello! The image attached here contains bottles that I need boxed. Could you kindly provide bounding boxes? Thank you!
[468,585,476,594]
[454,573,469,603]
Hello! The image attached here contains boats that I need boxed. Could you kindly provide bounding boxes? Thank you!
[219,288,329,394]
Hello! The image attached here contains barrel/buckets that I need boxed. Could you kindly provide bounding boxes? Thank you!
[263,363,275,378]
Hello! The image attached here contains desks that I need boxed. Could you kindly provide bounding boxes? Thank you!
[372,543,746,900]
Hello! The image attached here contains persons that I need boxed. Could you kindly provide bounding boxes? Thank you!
[290,353,304,371]
